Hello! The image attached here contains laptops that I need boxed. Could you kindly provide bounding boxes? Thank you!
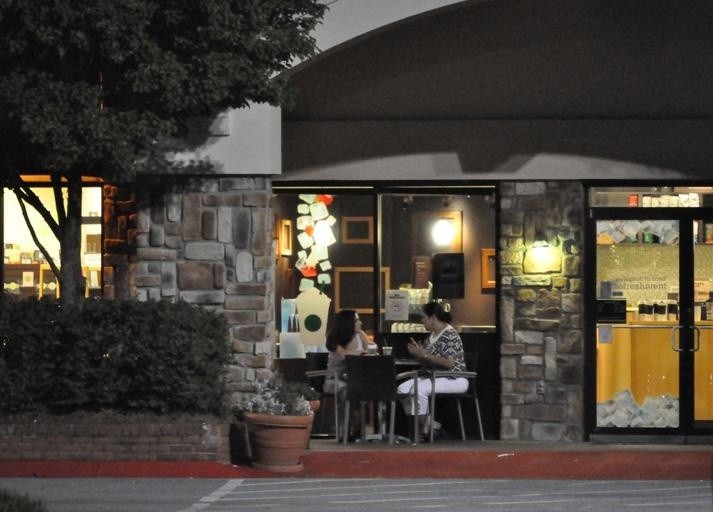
[597,300,626,323]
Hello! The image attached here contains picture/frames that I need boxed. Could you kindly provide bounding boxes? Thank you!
[332,266,390,314]
[409,211,463,254]
[341,217,374,244]
[280,218,294,258]
[480,248,497,290]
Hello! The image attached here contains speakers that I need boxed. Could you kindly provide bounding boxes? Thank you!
[433,253,465,300]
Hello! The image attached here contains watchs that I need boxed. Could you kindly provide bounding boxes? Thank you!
[423,352,429,362]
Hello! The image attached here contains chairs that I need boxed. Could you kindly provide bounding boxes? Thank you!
[305,350,486,446]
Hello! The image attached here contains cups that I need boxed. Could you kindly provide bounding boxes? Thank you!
[368,345,377,356]
[382,347,392,356]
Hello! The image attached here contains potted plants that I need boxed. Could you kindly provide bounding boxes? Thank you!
[240,372,320,472]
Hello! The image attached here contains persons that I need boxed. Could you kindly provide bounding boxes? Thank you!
[323,309,369,441]
[395,301,470,445]
